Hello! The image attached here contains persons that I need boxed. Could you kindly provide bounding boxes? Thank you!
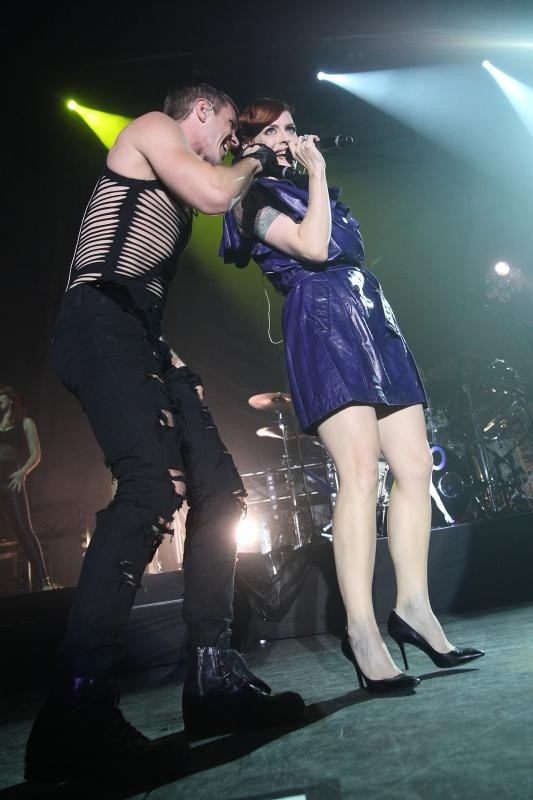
[218,99,486,694]
[25,82,305,788]
[0,385,52,593]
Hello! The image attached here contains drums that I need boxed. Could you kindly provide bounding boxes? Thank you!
[331,457,387,509]
[383,440,475,529]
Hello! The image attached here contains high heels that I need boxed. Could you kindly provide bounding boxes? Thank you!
[387,611,485,669]
[340,622,420,696]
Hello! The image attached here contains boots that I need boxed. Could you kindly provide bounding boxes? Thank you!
[21,669,191,783]
[177,638,304,741]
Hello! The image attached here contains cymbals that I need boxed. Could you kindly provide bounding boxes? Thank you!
[248,393,292,413]
[427,354,496,378]
[256,424,316,440]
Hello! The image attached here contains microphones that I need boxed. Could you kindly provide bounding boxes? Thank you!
[270,165,293,180]
[286,135,354,163]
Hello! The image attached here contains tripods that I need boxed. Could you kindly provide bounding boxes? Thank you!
[455,395,533,523]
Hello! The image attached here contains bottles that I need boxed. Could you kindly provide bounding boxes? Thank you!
[148,549,159,574]
[260,522,271,554]
